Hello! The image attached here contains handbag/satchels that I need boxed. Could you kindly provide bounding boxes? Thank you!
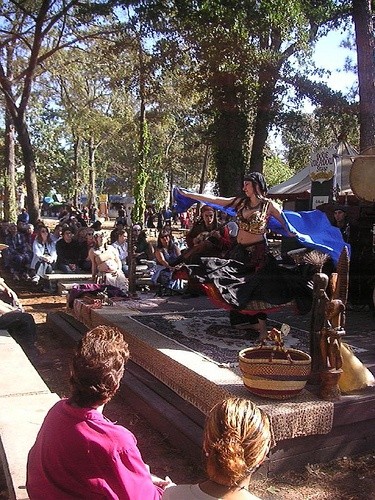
[238,331,312,401]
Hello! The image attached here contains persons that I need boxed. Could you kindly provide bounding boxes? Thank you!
[0,244,35,344]
[26,325,170,500]
[145,205,178,229]
[89,228,129,292]
[330,204,351,239]
[152,229,184,290]
[182,205,230,299]
[18,208,30,224]
[154,171,351,330]
[162,397,271,500]
[0,221,148,292]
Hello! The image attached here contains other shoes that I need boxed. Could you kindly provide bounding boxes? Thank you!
[32,276,39,286]
[22,274,31,281]
[13,273,20,280]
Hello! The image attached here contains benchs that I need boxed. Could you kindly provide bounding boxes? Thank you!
[0,330,65,500]
[42,273,94,296]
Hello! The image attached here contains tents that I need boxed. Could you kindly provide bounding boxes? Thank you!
[266,138,359,212]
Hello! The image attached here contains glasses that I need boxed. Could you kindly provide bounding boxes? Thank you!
[161,234,168,238]
